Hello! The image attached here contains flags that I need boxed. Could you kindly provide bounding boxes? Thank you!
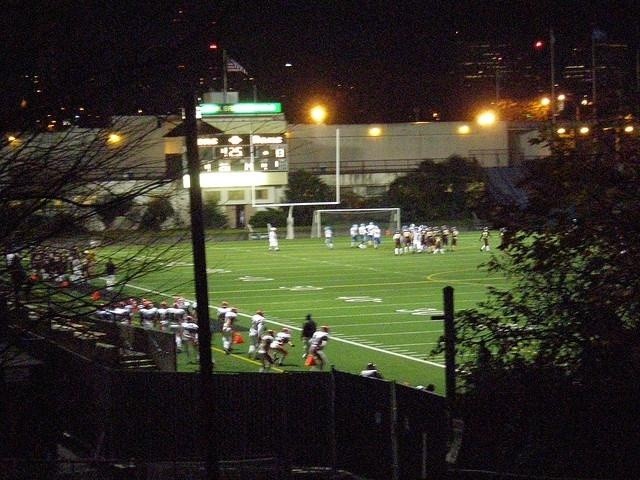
[225,55,249,76]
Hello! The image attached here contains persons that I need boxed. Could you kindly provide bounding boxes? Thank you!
[238,210,245,227]
[4,244,117,302]
[416,383,438,393]
[349,221,382,250]
[268,228,280,251]
[360,362,384,379]
[479,227,492,251]
[392,223,459,256]
[323,225,335,249]
[93,295,331,373]
